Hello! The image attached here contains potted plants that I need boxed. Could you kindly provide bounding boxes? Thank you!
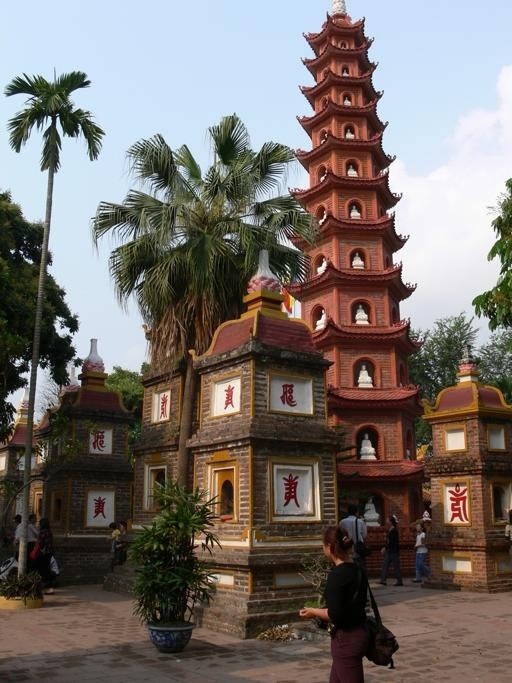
[126,471,225,654]
[0,572,48,609]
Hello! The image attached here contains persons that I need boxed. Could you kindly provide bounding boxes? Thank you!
[356,364,373,383]
[363,496,381,522]
[105,521,121,553]
[355,303,368,319]
[13,513,22,562]
[359,433,376,455]
[505,509,512,555]
[349,204,362,217]
[30,516,55,595]
[111,522,127,572]
[339,504,369,604]
[299,525,371,682]
[351,250,364,266]
[348,163,358,175]
[27,513,41,568]
[373,516,403,586]
[316,308,327,325]
[409,522,431,582]
[317,255,326,272]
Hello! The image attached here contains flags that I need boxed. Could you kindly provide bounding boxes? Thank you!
[282,275,296,312]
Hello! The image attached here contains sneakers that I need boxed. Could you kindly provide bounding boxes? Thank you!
[376,580,422,586]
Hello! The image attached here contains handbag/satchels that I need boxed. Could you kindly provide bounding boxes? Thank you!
[354,539,373,557]
[363,615,399,666]
